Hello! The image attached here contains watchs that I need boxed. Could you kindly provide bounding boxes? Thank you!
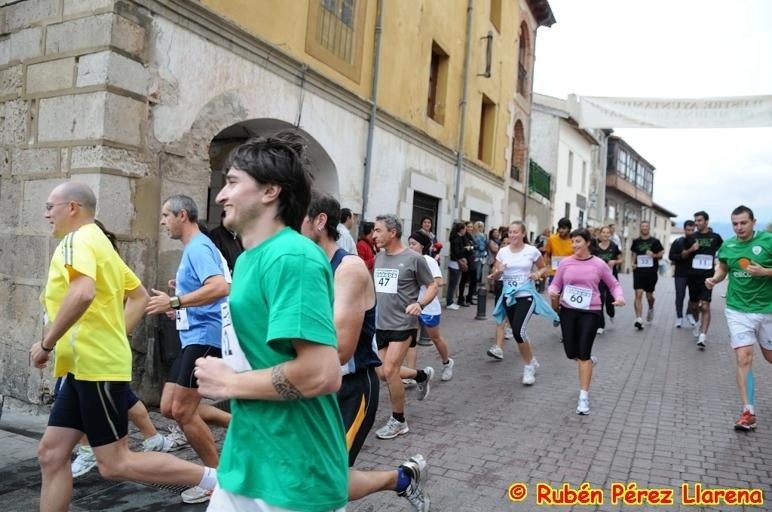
[416,301,424,310]
[170,296,181,310]
[416,300,425,311]
[41,337,53,352]
[40,338,54,353]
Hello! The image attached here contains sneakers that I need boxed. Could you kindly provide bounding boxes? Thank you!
[472,296,478,299]
[687,316,696,326]
[503,328,513,339]
[576,397,590,415]
[401,379,417,388]
[140,435,173,453]
[693,325,701,337]
[416,367,434,401]
[696,335,706,349]
[610,316,615,322]
[591,356,598,368]
[440,359,454,381]
[376,416,409,439]
[487,345,503,359]
[71,446,98,477]
[634,319,644,330]
[647,309,656,321]
[522,358,539,385]
[446,303,460,310]
[676,319,683,328]
[734,408,757,430]
[554,320,559,327]
[166,425,192,451]
[397,454,430,512]
[596,327,605,334]
[181,486,213,503]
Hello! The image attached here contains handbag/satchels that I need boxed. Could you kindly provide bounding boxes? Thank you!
[453,254,468,272]
[487,245,495,265]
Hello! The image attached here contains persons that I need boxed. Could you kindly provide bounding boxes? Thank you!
[209,211,245,274]
[30,180,216,511]
[446,222,468,311]
[546,228,627,415]
[194,129,350,512]
[535,227,551,256]
[488,234,512,361]
[487,360,492,360]
[500,226,509,237]
[356,220,380,275]
[630,220,664,331]
[474,221,488,305]
[486,228,499,297]
[408,230,454,382]
[431,242,443,266]
[372,213,439,439]
[681,210,723,347]
[705,205,772,431]
[543,218,576,327]
[71,219,174,477]
[668,220,696,328]
[416,217,442,266]
[597,226,626,334]
[169,220,233,290]
[488,220,560,385]
[457,222,480,309]
[300,187,430,510]
[488,229,500,268]
[144,194,233,504]
[608,224,622,253]
[487,236,520,360]
[336,208,358,256]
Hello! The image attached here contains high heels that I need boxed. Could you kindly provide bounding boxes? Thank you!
[458,297,470,306]
[466,296,476,305]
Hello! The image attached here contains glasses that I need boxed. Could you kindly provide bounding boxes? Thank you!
[46,202,82,210]
